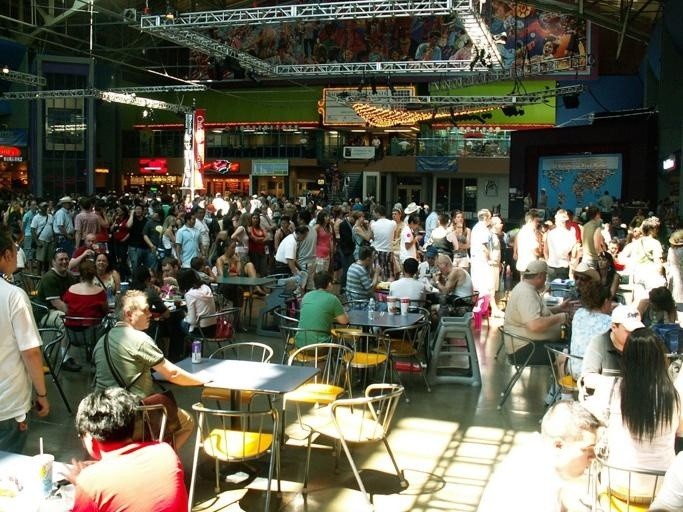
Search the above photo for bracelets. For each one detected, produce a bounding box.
[35,391,48,397]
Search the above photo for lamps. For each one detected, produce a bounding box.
[200,52,266,85]
[356,47,526,132]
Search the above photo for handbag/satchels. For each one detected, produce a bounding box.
[215,318,233,338]
[155,247,171,263]
[140,389,182,442]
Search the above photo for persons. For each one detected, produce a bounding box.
[386,258,427,342]
[1,191,508,319]
[478,259,681,512]
[426,255,474,339]
[398,139,411,151]
[57,386,189,512]
[513,189,683,326]
[372,135,380,148]
[35,249,217,453]
[190,2,584,80]
[345,245,384,338]
[1,229,50,455]
[290,271,350,385]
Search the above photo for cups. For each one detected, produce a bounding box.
[396,299,400,314]
[386,296,396,315]
[172,296,180,309]
[119,283,128,294]
[31,452,54,493]
[400,297,410,316]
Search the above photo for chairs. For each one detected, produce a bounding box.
[537,260,678,508]
[2,251,322,512]
[290,383,409,497]
[283,250,490,431]
[495,324,563,409]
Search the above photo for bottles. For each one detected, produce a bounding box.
[367,298,375,320]
[115,291,121,306]
[560,323,567,344]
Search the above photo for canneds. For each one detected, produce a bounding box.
[192,341,201,364]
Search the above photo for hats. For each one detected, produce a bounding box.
[56,196,75,206]
[522,260,554,276]
[426,247,438,258]
[393,203,403,214]
[403,202,421,214]
[669,228,683,246]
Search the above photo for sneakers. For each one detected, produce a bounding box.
[61,358,80,372]
